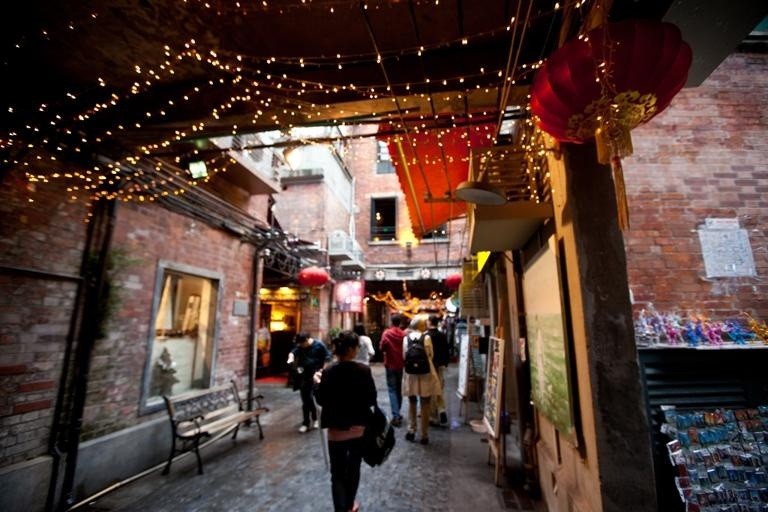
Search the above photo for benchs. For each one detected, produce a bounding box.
[163,375,270,474]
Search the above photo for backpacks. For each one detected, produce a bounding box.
[404,338,430,374]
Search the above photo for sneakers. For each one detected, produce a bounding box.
[312,419,320,429]
[391,418,402,428]
[298,425,309,432]
[421,438,428,445]
[405,432,415,441]
[428,408,448,427]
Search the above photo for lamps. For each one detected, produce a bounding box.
[456,157,528,205]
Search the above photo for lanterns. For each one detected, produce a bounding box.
[297,264,330,297]
[528,13,694,234]
[445,274,462,291]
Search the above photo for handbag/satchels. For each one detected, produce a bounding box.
[286,356,308,392]
[361,407,395,468]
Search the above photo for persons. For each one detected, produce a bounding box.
[287,330,331,436]
[309,330,378,512]
[421,315,451,432]
[351,322,375,371]
[380,312,407,429]
[397,315,436,446]
